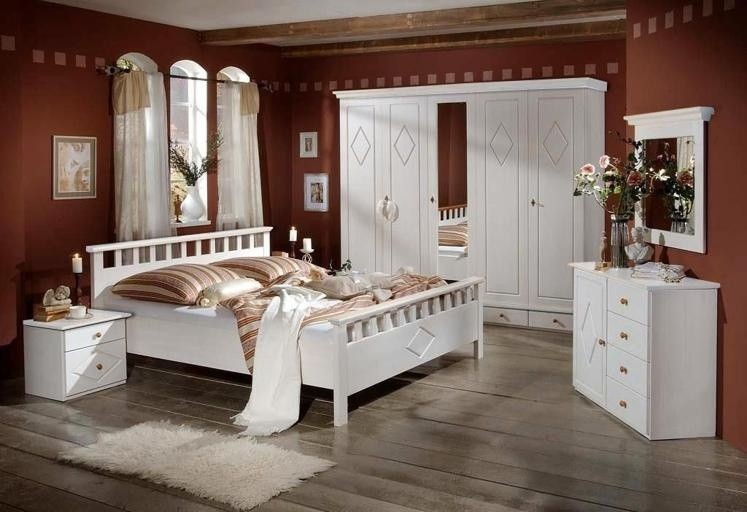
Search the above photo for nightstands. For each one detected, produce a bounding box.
[21,306,131,400]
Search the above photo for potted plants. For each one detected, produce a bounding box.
[165,127,225,223]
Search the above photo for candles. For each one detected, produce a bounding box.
[302,237,312,251]
[289,226,297,241]
[71,252,82,274]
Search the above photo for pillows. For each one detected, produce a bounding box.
[303,278,369,300]
[456,219,467,228]
[209,255,330,288]
[437,224,467,247]
[111,260,247,305]
[199,276,262,310]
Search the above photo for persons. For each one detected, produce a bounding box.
[49,285,72,305]
[314,183,321,202]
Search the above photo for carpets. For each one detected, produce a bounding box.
[59,420,337,512]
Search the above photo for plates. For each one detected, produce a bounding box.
[65,313,94,321]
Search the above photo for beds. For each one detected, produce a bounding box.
[437,203,468,277]
[84,224,485,428]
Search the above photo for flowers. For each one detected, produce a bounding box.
[649,137,697,218]
[307,257,352,283]
[562,128,675,265]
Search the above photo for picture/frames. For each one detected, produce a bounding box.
[303,172,329,213]
[51,134,98,201]
[299,131,318,159]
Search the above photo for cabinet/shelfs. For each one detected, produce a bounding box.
[332,87,437,276]
[568,262,721,440]
[477,77,608,333]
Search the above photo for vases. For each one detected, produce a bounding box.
[610,214,629,267]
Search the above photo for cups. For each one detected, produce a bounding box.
[69,306,87,318]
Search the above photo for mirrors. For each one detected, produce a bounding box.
[620,104,716,255]
[438,102,468,285]
